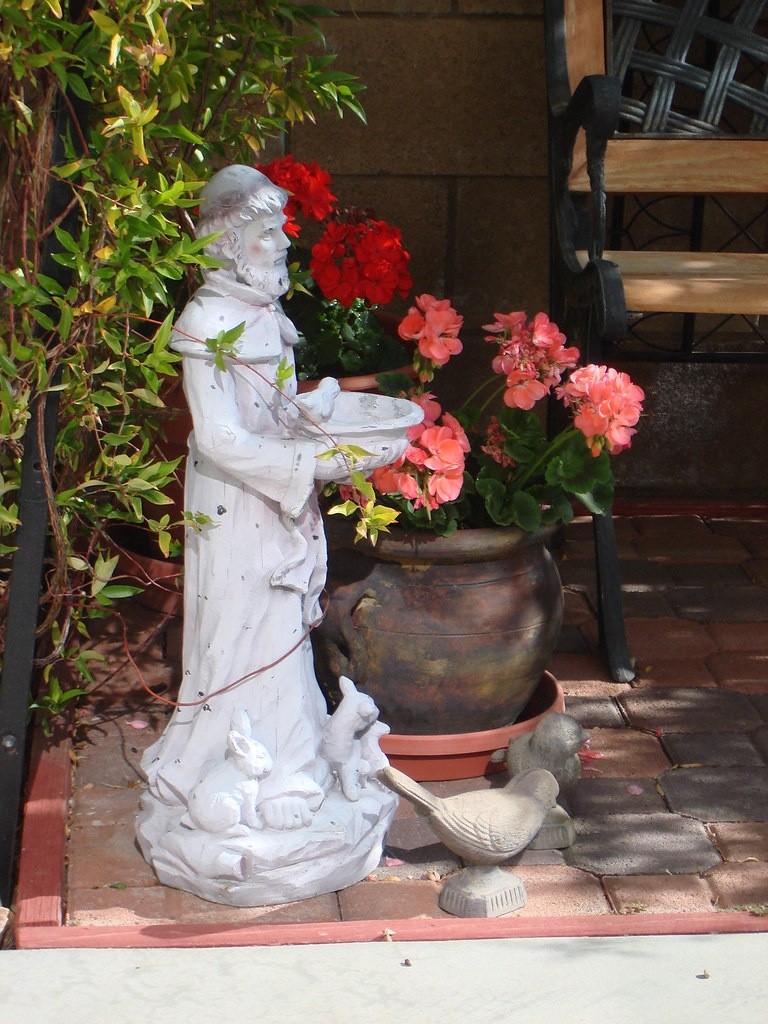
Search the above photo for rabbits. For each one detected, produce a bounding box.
[180,707,274,837]
[313,676,390,802]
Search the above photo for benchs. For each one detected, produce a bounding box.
[537,0,768,682]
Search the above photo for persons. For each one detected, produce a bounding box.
[133,165,425,907]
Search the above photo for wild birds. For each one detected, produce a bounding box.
[492,713,591,792]
[374,764,571,869]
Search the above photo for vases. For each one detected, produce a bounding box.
[93,314,417,547]
[323,521,570,726]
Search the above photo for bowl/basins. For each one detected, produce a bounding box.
[289,391,425,469]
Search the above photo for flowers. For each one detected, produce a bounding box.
[239,157,415,395]
[322,297,642,537]
